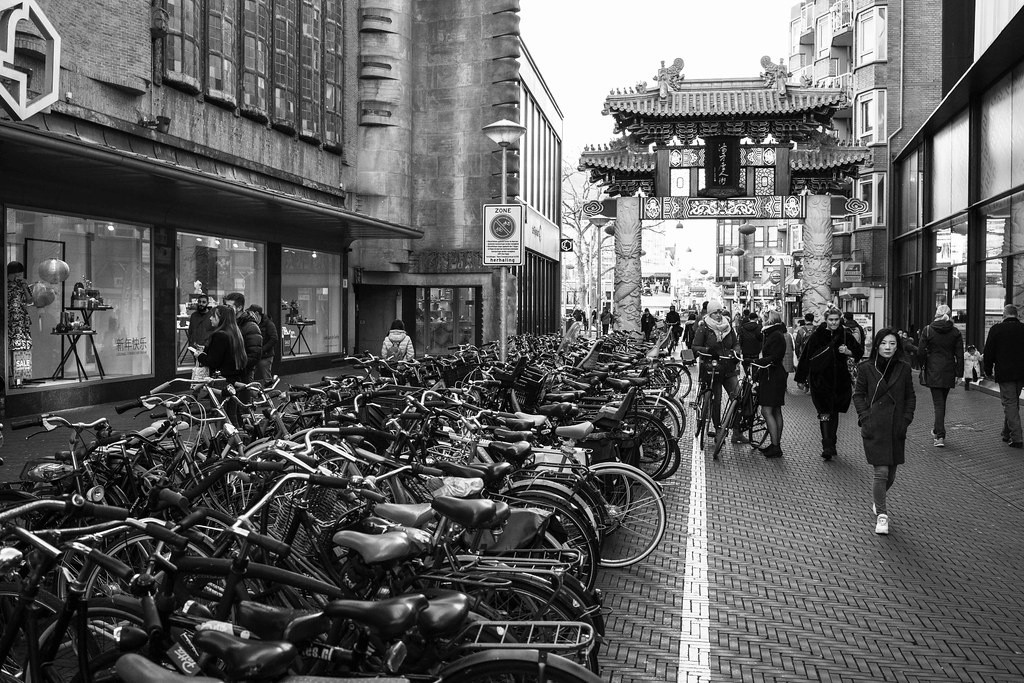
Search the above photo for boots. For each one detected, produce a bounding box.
[819,420,832,459]
[831,419,837,456]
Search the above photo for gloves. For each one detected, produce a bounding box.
[731,353,741,364]
[708,349,719,362]
[742,359,756,369]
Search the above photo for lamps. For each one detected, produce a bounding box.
[139,116,172,134]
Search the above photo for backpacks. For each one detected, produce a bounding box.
[385,338,405,362]
[799,326,816,353]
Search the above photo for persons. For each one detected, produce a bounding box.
[592,310,597,324]
[918,304,983,447]
[193,305,248,429]
[985,304,1024,447]
[7,261,34,389]
[245,304,278,407]
[600,307,613,335]
[641,308,656,340]
[665,306,680,345]
[381,320,415,385]
[795,307,919,533]
[683,300,794,456]
[223,293,263,404]
[188,296,212,364]
[582,311,588,331]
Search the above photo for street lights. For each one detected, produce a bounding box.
[590,212,612,340]
[479,117,526,363]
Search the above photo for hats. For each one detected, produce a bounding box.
[706,300,722,315]
[245,304,262,313]
[7,260,23,274]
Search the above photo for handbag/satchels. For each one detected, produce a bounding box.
[919,366,928,386]
[190,356,209,390]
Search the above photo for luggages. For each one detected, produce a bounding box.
[680,340,696,366]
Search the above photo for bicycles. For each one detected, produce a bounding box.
[0,322,693,683]
[795,352,857,398]
[707,351,770,460]
[689,350,738,452]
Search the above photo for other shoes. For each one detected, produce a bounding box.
[931,429,937,440]
[1003,437,1010,442]
[1008,441,1022,448]
[758,444,783,458]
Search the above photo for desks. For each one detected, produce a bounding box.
[51,305,112,381]
[287,322,315,354]
[176,326,197,364]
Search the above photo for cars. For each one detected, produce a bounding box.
[952,294,968,322]
[985,284,1006,316]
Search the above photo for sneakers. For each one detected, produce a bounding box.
[875,514,889,532]
[934,438,944,447]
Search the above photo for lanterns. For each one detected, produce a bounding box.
[37,257,69,293]
[605,225,615,236]
[566,263,574,269]
[732,248,745,256]
[701,270,719,287]
[739,224,756,236]
[726,267,738,282]
[26,281,55,333]
[640,250,646,256]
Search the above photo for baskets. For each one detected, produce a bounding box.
[274,483,366,563]
[456,363,484,387]
[372,395,407,418]
